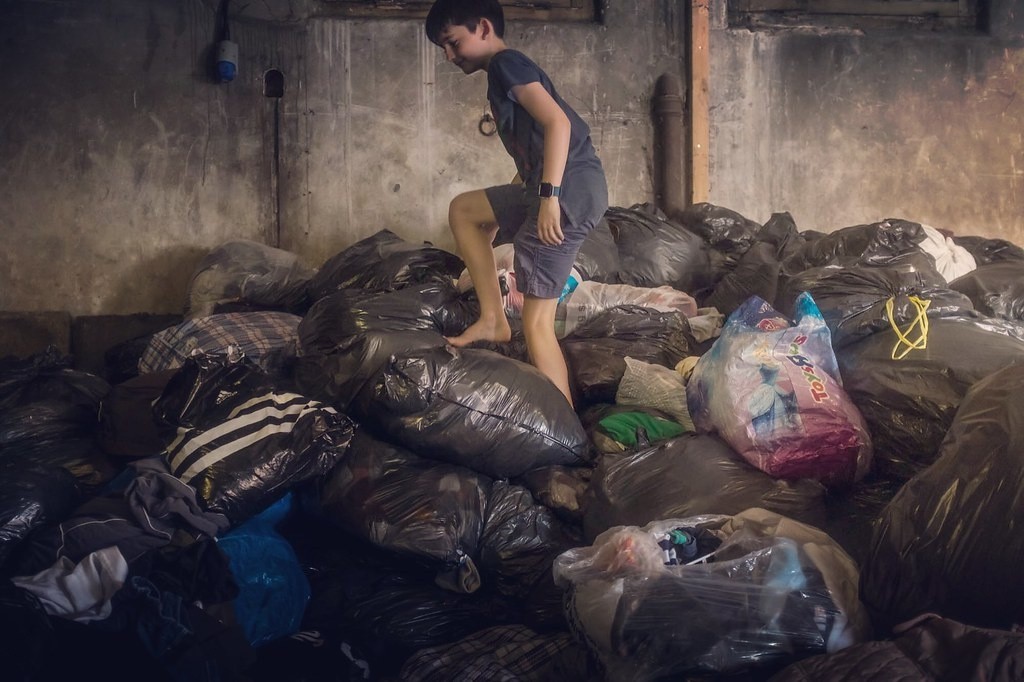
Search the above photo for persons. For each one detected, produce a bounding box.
[425,0,608,409]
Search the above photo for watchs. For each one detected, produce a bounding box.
[538,183,563,197]
